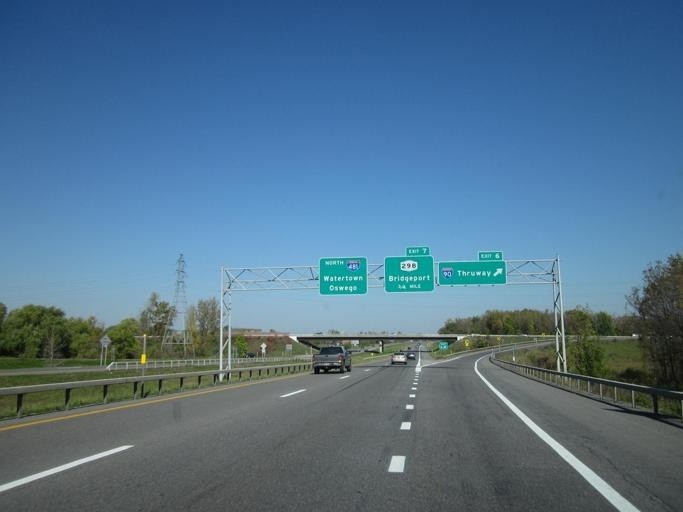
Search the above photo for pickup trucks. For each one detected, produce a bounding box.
[312,345,352,374]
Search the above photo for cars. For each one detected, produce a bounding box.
[390,351,408,365]
[407,352,416,360]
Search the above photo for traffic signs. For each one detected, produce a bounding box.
[383,255,436,294]
[319,256,368,297]
[438,260,508,286]
